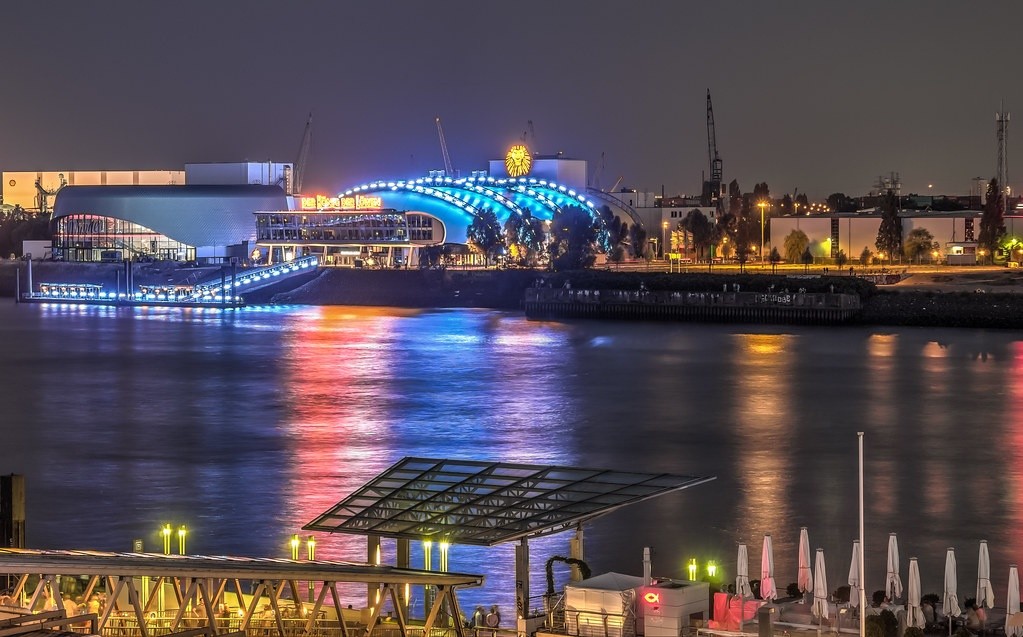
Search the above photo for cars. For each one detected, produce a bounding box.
[180,259,199,268]
[669,258,678,263]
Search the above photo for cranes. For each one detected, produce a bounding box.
[436,117,452,176]
[610,176,623,192]
[706,88,723,182]
[593,152,604,187]
[293,112,312,194]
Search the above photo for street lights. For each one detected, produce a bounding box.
[663,222,668,261]
[424,540,432,622]
[707,560,715,577]
[308,536,314,603]
[439,542,447,572]
[162,524,171,555]
[291,534,298,599]
[688,558,696,581]
[758,202,767,268]
[178,524,186,555]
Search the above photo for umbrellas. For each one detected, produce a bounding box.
[760,534,777,605]
[848,539,867,620]
[906,557,926,630]
[886,532,903,605]
[798,527,813,604]
[811,547,829,630]
[735,542,754,599]
[976,539,995,637]
[942,547,961,637]
[1005,563,1020,637]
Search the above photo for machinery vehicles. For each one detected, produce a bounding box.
[136,251,154,263]
[43,251,64,262]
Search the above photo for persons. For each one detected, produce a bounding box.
[62,595,77,616]
[192,598,206,626]
[88,596,99,613]
[965,604,987,637]
[920,601,934,623]
[222,604,230,633]
[720,584,734,593]
[880,596,889,608]
[475,605,501,628]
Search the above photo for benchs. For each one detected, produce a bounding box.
[779,602,813,625]
